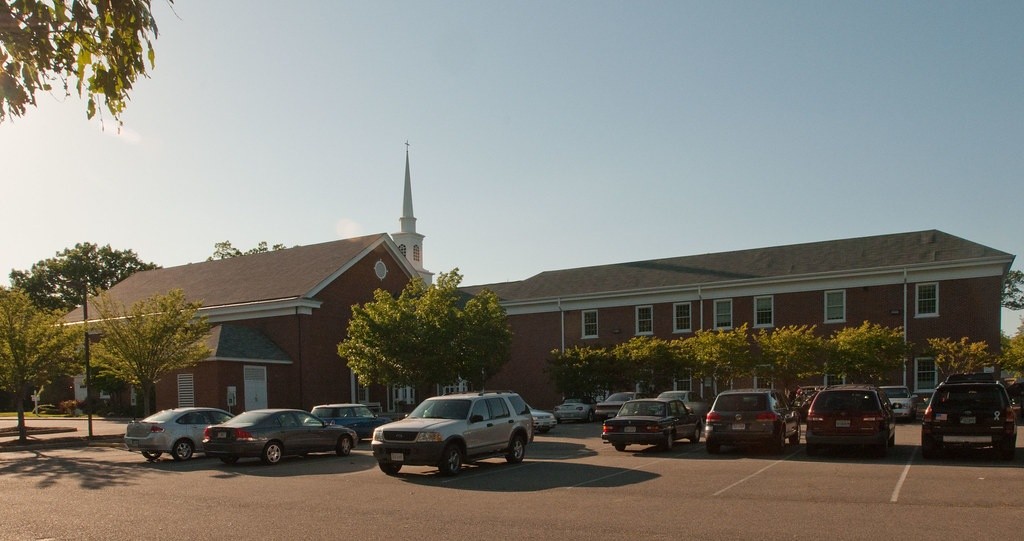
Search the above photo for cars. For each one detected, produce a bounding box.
[124,406,236,463]
[654,390,711,421]
[913,389,937,422]
[788,385,825,422]
[601,398,702,454]
[306,402,393,441]
[555,395,598,420]
[526,401,557,434]
[595,391,651,423]
[201,408,360,466]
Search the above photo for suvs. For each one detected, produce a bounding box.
[921,373,1019,461]
[805,384,903,456]
[704,388,800,455]
[878,385,919,424]
[371,389,535,477]
